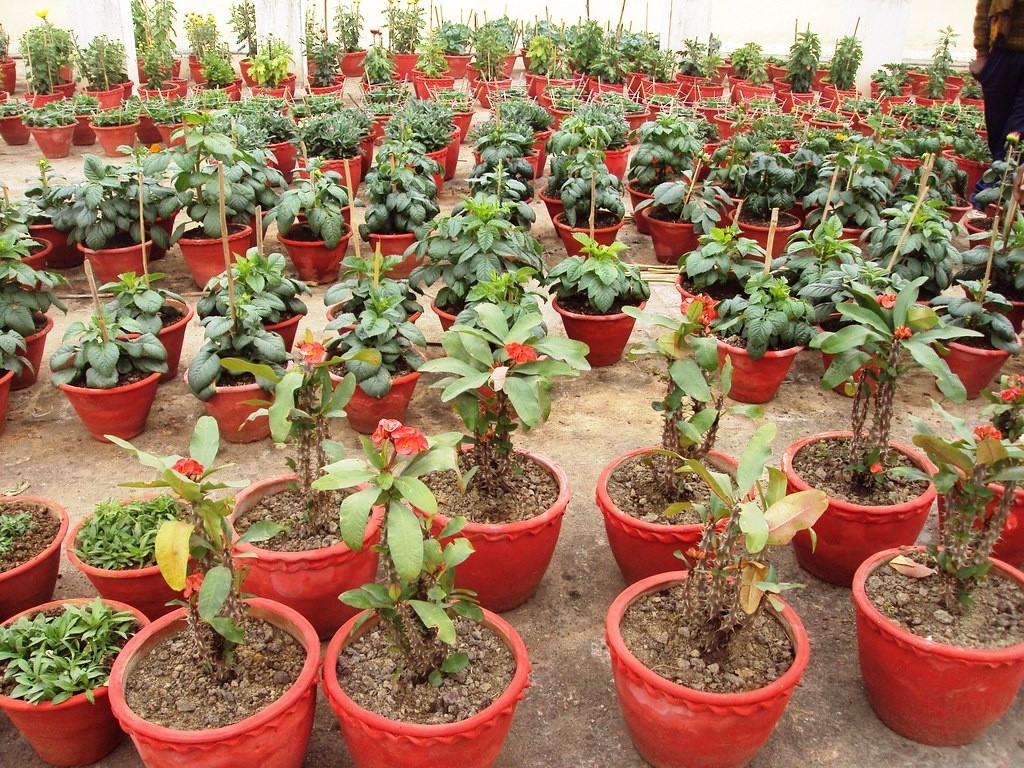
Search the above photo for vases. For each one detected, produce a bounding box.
[780,432,942,587]
[234,78,243,101]
[106,595,323,768]
[121,80,134,101]
[81,83,125,107]
[338,49,368,77]
[322,599,533,768]
[937,436,1024,569]
[188,54,198,79]
[23,89,64,108]
[301,81,344,96]
[391,73,401,80]
[393,54,420,81]
[362,80,389,91]
[225,471,388,639]
[137,83,180,102]
[37,79,76,97]
[931,333,1023,399]
[189,63,206,84]
[852,544,1024,748]
[410,440,571,611]
[192,82,237,100]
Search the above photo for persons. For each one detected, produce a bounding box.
[969,0,1024,163]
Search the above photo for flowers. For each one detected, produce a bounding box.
[134,33,179,90]
[369,32,399,77]
[99,122,1024,689]
[186,17,239,81]
[65,27,121,91]
[331,0,365,52]
[380,0,405,52]
[391,0,428,54]
[95,35,130,84]
[298,0,324,57]
[29,6,79,84]
[17,21,70,93]
[358,28,397,84]
[314,28,340,87]
[199,43,236,88]
[182,11,222,54]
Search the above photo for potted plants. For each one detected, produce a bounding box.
[799,255,922,396]
[0,597,152,768]
[540,232,652,367]
[0,231,73,389]
[70,493,208,621]
[0,0,1024,274]
[186,300,299,445]
[194,246,313,369]
[604,421,831,768]
[321,285,431,437]
[676,222,772,333]
[952,228,1024,337]
[320,254,425,337]
[595,301,757,589]
[88,268,193,382]
[0,495,69,624]
[705,272,821,404]
[48,301,171,442]
[402,195,548,352]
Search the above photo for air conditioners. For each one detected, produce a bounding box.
[0,329,35,434]
